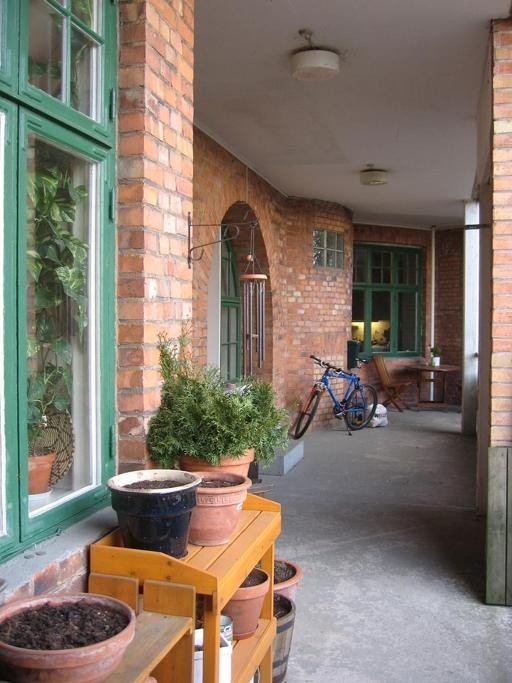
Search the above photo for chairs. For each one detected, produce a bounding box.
[371,355,411,412]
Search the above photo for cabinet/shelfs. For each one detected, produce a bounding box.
[88,488,281,682]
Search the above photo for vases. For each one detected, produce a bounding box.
[0,589,135,683]
[192,559,302,683]
[108,469,252,558]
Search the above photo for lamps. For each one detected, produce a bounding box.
[289,30,342,82]
[360,164,390,186]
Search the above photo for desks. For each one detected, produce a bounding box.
[406,365,460,408]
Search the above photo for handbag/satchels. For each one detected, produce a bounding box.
[365,403,388,427]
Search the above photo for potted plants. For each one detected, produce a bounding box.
[429,345,442,367]
[27,0,92,495]
[147,313,306,477]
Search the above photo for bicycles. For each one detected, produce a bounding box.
[294,354,378,438]
